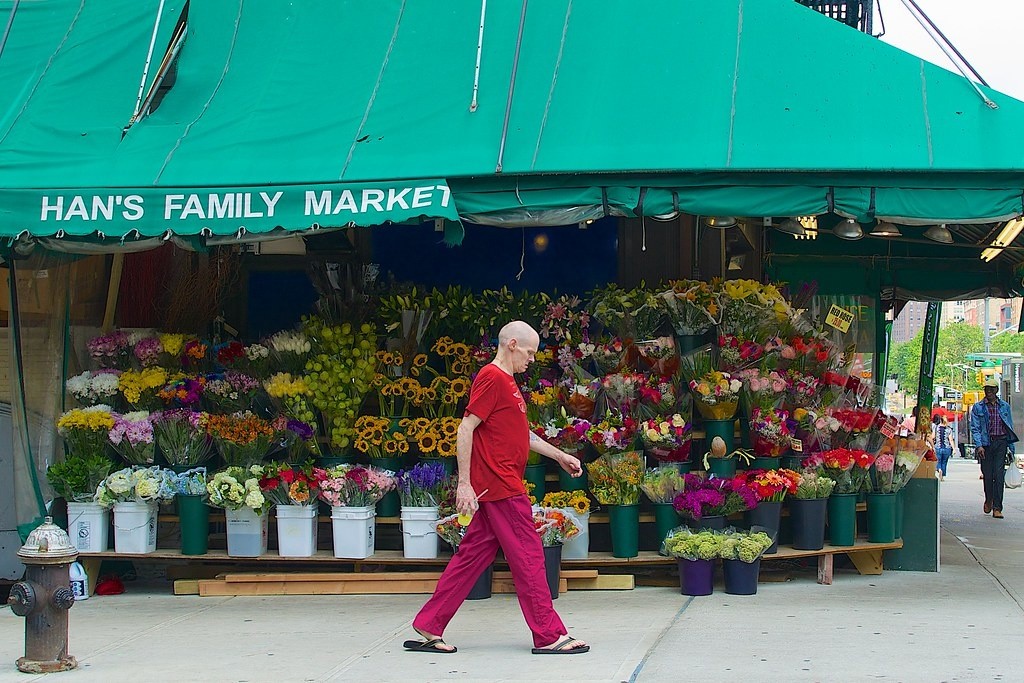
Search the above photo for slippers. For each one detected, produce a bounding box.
[403,639,457,653]
[532,637,590,654]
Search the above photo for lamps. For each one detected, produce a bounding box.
[703,215,738,229]
[922,224,954,244]
[981,216,1024,262]
[832,216,866,240]
[869,221,902,236]
[773,215,808,236]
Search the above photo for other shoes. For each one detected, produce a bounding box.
[984,497,993,513]
[939,469,945,482]
[993,508,1004,518]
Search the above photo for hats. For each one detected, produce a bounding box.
[984,378,999,389]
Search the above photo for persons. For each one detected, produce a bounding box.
[403,318,592,654]
[959,417,970,457]
[931,413,956,483]
[970,378,1020,518]
[899,406,918,434]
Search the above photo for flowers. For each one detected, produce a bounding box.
[55,276,930,563]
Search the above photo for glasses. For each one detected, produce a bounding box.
[984,387,997,393]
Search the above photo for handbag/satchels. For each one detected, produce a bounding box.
[1004,462,1021,489]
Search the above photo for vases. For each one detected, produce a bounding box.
[824,493,860,546]
[742,501,783,555]
[453,545,493,600]
[523,464,547,504]
[417,455,454,481]
[175,493,208,555]
[316,455,350,517]
[720,556,762,595]
[365,456,404,517]
[606,502,640,557]
[556,463,589,496]
[697,513,729,531]
[166,464,200,476]
[651,503,685,556]
[543,541,564,599]
[865,493,897,543]
[674,556,716,596]
[786,497,828,550]
[702,417,738,456]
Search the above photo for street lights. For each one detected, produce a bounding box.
[945,364,976,392]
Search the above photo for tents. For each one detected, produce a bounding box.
[930,407,964,424]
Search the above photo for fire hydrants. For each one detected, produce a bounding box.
[7,516,83,674]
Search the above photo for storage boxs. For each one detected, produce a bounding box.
[910,461,938,479]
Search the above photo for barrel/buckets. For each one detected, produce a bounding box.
[111,501,160,554]
[65,501,109,553]
[531,505,590,560]
[276,501,319,557]
[329,504,378,560]
[400,505,441,559]
[225,504,268,557]
